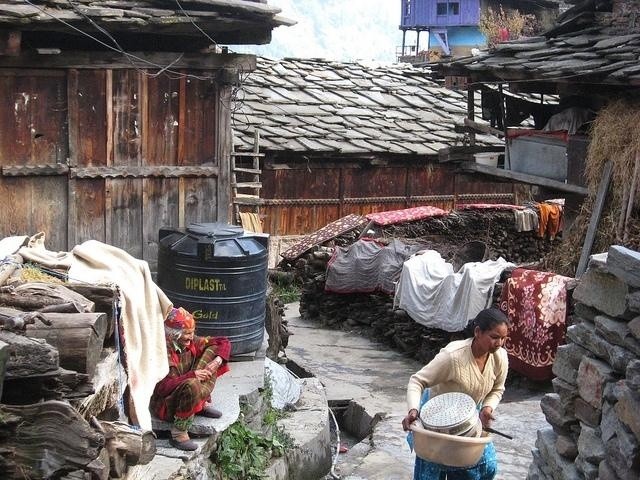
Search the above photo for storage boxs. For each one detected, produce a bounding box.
[505,128,591,186]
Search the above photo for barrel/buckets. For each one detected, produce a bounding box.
[474,152,499,169]
[158,221,270,356]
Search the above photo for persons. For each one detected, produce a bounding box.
[403,307,509,480]
[148,305,232,451]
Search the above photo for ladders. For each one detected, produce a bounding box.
[230,128,265,228]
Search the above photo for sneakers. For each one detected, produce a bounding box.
[168,433,198,451]
[196,406,222,418]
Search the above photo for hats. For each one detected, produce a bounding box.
[165,306,196,330]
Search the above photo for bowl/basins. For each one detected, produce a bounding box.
[407,421,495,466]
